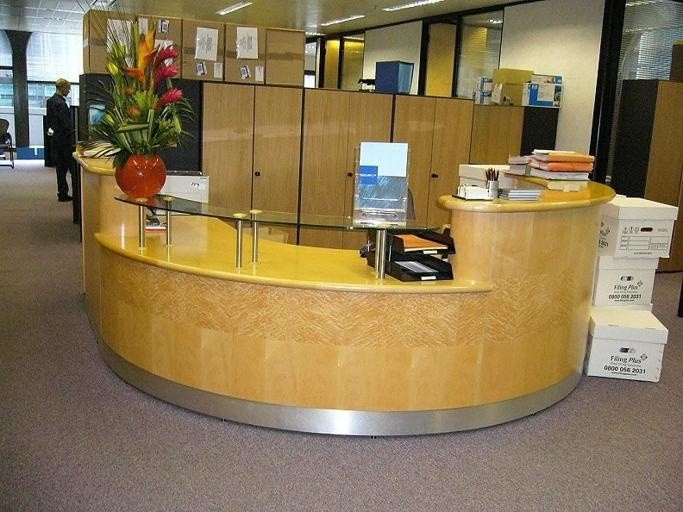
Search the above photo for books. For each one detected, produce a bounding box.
[498,147,596,202]
[144,217,166,233]
[391,232,448,252]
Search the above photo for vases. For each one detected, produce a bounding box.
[113,155,167,201]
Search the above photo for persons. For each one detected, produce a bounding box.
[41,77,79,203]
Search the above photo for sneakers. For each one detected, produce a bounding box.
[57,193,73,202]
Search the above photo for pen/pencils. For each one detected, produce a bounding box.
[486,168,499,181]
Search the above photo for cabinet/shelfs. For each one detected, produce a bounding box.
[469,103,524,166]
[298,89,394,252]
[200,81,303,249]
[392,91,474,237]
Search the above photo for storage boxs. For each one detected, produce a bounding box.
[470,68,565,110]
[581,195,678,385]
[81,8,306,87]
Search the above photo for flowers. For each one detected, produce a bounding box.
[76,1,198,165]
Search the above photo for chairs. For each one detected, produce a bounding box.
[0,117,14,168]
